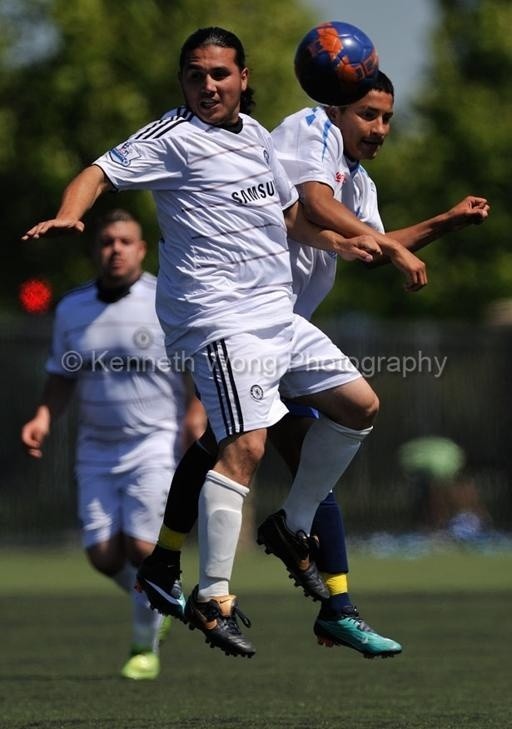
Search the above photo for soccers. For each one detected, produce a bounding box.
[294,22,378,108]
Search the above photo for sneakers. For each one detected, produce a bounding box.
[134,545,187,620]
[313,608,403,659]
[255,507,331,602]
[120,616,174,681]
[183,583,257,659]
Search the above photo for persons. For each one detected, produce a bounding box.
[15,25,387,658]
[131,61,493,660]
[15,207,207,684]
